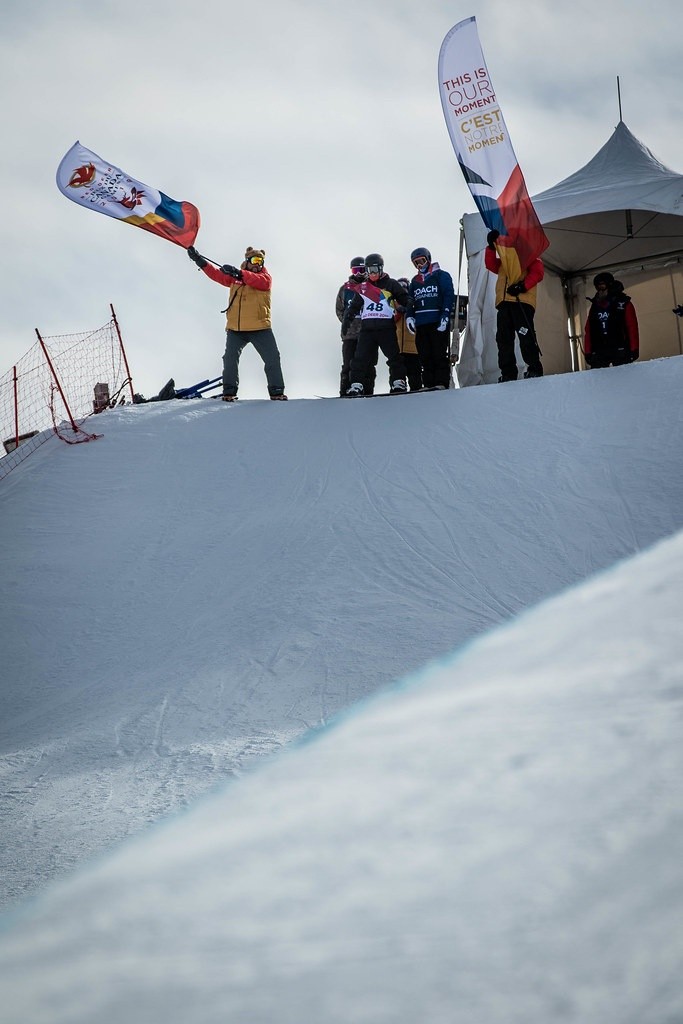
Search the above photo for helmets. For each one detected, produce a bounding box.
[410,247,432,274]
[364,253,384,267]
[349,256,366,269]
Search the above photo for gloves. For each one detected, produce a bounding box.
[486,229,500,250]
[506,280,527,297]
[436,317,449,331]
[584,352,595,366]
[672,304,683,317]
[629,350,640,361]
[342,318,351,337]
[405,317,416,335]
[219,264,243,281]
[187,245,207,268]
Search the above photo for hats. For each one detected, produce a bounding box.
[397,277,410,290]
[593,272,614,287]
[240,247,266,270]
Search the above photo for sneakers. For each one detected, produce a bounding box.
[391,379,406,392]
[345,383,364,396]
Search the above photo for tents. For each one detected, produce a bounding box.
[449,121,683,389]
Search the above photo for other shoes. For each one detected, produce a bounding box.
[222,395,239,402]
[270,394,288,401]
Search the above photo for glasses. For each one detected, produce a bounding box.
[367,264,384,274]
[244,255,264,266]
[397,281,408,288]
[351,266,367,275]
[595,277,612,291]
[412,255,431,269]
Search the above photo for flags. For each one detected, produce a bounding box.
[437,17,550,285]
[55,142,200,248]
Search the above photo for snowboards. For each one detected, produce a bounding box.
[313,385,445,399]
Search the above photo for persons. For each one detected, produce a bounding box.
[335,257,367,396]
[485,230,544,383]
[187,246,288,401]
[404,248,454,390]
[583,272,640,370]
[342,253,408,394]
[393,278,423,390]
